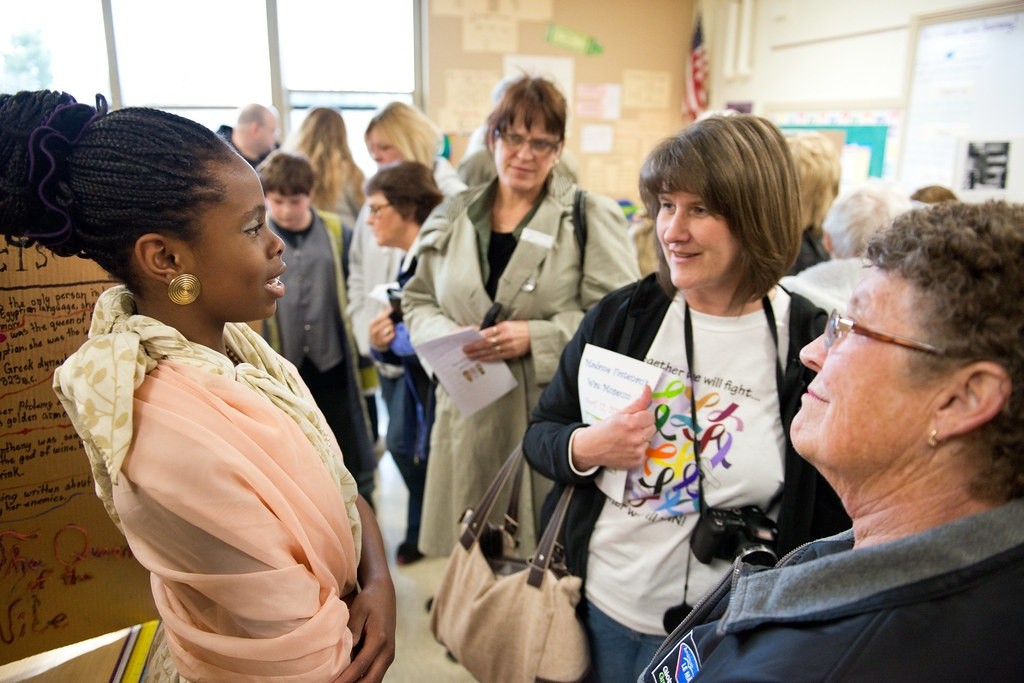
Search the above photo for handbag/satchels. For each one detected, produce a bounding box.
[427,440,592,683]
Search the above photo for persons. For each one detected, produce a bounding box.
[261,108,367,220]
[399,79,640,559]
[786,130,956,320]
[218,103,280,168]
[348,102,468,566]
[522,115,852,683]
[637,196,1024,683]
[246,152,377,513]
[0,91,396,683]
[458,74,578,187]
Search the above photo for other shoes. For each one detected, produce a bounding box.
[397,540,426,564]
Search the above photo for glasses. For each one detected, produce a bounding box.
[824,310,941,358]
[495,129,560,156]
[369,203,393,218]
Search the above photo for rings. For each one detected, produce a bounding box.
[496,345,500,351]
[492,338,497,344]
[384,329,389,334]
[493,327,496,335]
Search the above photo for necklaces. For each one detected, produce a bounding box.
[225,346,242,365]
